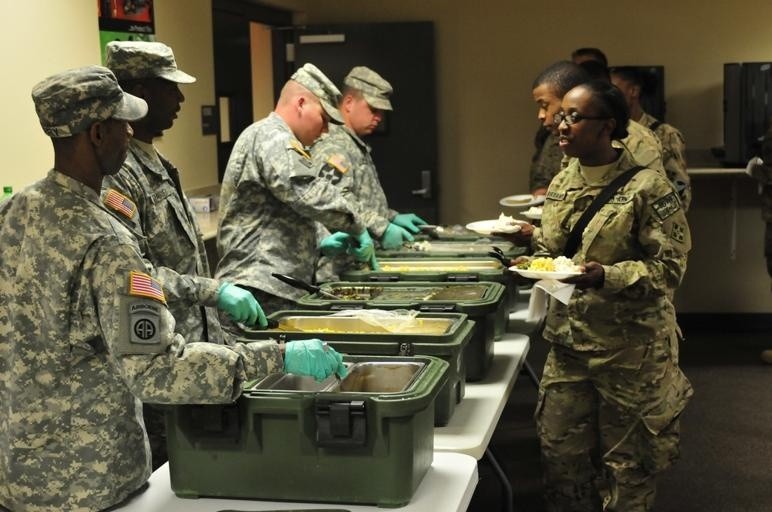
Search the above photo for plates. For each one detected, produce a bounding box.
[497,190,547,208]
[463,216,529,238]
[521,209,543,220]
[508,264,587,284]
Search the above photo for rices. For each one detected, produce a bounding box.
[552,255,576,273]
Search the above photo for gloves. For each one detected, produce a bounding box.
[352,230,376,270]
[320,232,352,256]
[380,223,414,250]
[392,213,428,233]
[284,338,347,382]
[217,281,268,329]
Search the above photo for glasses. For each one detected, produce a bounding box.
[554,113,610,125]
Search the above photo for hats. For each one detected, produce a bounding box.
[344,66,394,111]
[32,67,149,138]
[290,63,345,125]
[106,41,196,84]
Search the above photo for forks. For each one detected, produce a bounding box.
[486,244,508,268]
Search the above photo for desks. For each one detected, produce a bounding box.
[431,331,541,512]
[103,449,481,512]
[503,289,548,345]
[194,210,218,244]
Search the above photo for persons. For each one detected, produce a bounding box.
[304,66,434,287]
[0,66,348,512]
[510,81,693,512]
[213,61,377,342]
[744,127,772,365]
[489,47,693,260]
[98,39,268,475]
